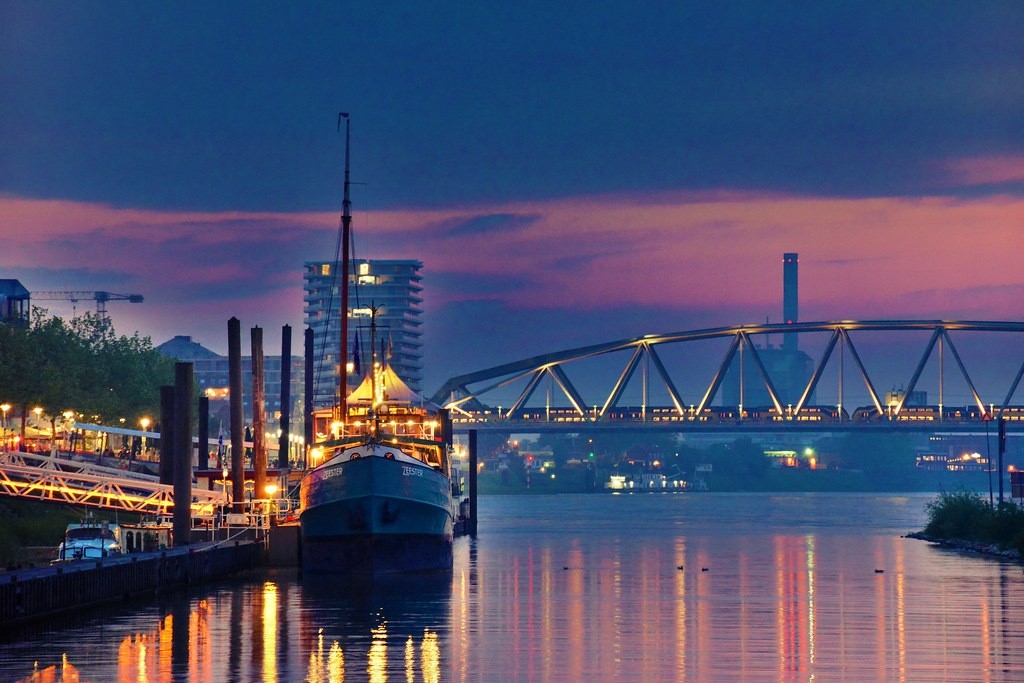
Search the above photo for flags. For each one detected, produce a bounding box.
[218,419,224,454]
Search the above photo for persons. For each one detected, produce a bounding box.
[94,447,115,458]
[118,449,130,459]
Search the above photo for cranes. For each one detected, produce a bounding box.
[28,289,144,330]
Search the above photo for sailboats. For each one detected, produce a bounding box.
[296,111,455,554]
